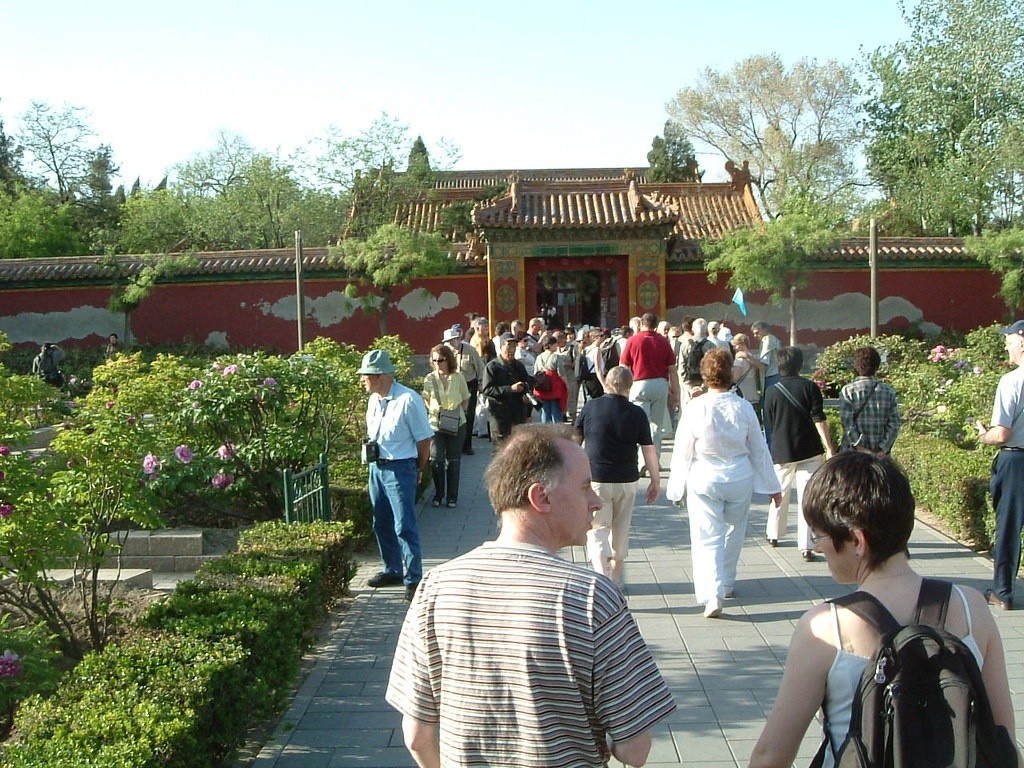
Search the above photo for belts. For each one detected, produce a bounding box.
[1001,447,1024,451]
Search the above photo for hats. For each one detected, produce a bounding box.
[707,321,721,331]
[999,320,1024,335]
[500,332,516,341]
[442,329,460,342]
[356,350,396,375]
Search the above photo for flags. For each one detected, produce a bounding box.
[733,288,747,316]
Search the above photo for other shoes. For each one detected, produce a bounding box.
[765,534,778,548]
[638,464,648,477]
[448,501,456,507]
[431,499,440,507]
[704,598,722,619]
[367,572,403,588]
[983,589,1013,611]
[405,583,418,601]
[467,450,473,455]
[802,551,816,562]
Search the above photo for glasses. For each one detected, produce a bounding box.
[432,359,446,363]
[672,336,679,338]
[809,528,865,544]
[523,338,530,343]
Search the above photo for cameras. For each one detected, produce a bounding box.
[361,439,377,464]
[521,382,530,392]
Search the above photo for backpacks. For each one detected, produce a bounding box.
[601,336,620,378]
[534,352,553,392]
[681,338,709,386]
[809,578,1019,768]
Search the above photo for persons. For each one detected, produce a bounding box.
[355,349,434,601]
[481,332,532,451]
[421,345,471,509]
[975,319,1024,610]
[622,313,679,477]
[452,312,643,439]
[384,422,677,768]
[666,348,782,618]
[838,347,901,459]
[32,343,66,392]
[748,450,1015,768]
[105,333,123,360]
[442,328,483,455]
[656,314,783,450]
[571,367,660,591]
[765,346,836,562]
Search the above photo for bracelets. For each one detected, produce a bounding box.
[746,355,752,361]
[417,468,423,472]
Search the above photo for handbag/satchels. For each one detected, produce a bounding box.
[438,409,461,436]
[583,371,604,400]
